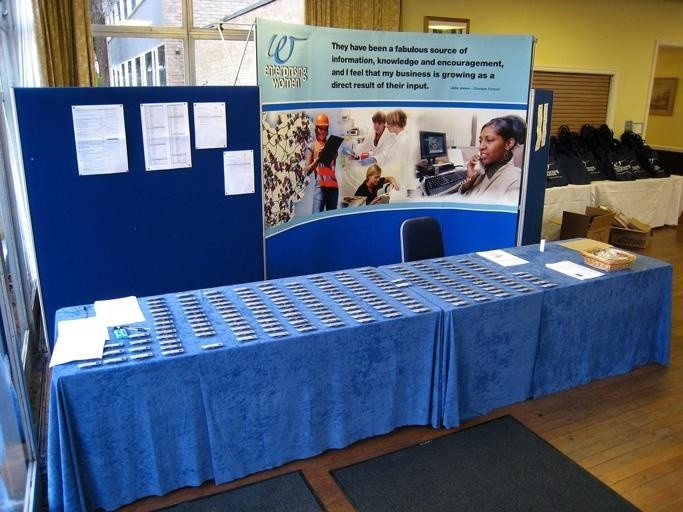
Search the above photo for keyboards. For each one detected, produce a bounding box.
[425,169,467,196]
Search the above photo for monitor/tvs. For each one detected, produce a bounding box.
[419,131,447,165]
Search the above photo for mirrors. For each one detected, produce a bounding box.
[642,42,682,153]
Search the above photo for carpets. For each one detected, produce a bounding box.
[151,468,329,512]
[329,415,641,512]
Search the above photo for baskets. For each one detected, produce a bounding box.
[581,247,636,272]
[609,223,652,249]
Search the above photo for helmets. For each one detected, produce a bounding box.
[315,114,329,126]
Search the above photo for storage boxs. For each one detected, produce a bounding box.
[551,207,616,244]
[610,218,652,249]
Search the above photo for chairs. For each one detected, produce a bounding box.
[400,217,444,263]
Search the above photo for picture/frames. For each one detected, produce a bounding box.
[649,78,679,116]
[424,16,470,33]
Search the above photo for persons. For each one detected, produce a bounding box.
[338,110,395,168]
[379,108,419,191]
[303,111,339,216]
[456,114,527,207]
[353,164,401,206]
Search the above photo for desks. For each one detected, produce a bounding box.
[541,174,683,242]
[47,266,444,512]
[377,237,673,429]
